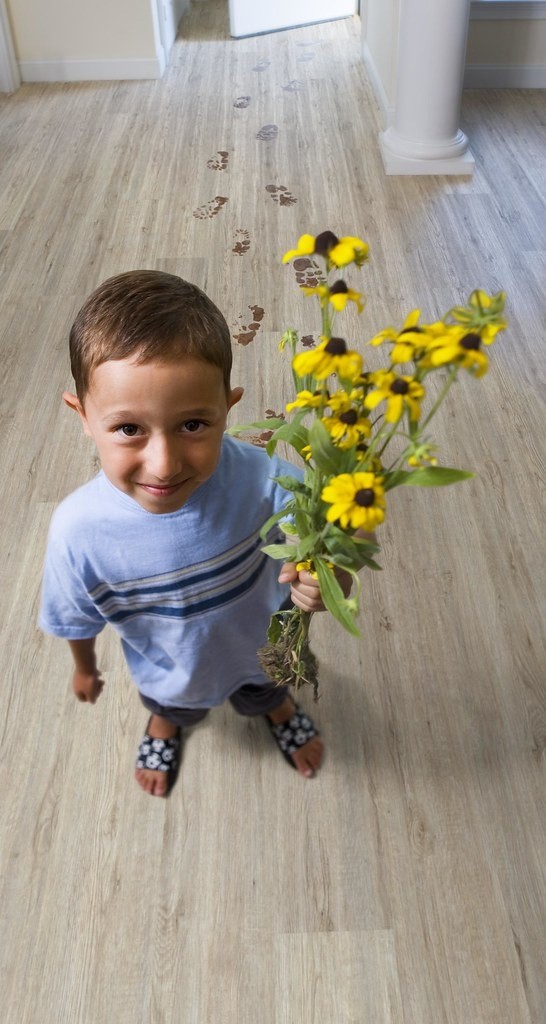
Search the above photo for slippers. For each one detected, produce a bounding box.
[136,714,182,797]
[264,696,321,770]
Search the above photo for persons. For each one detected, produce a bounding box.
[38,270,379,796]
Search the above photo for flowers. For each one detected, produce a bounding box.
[222,228,508,706]
